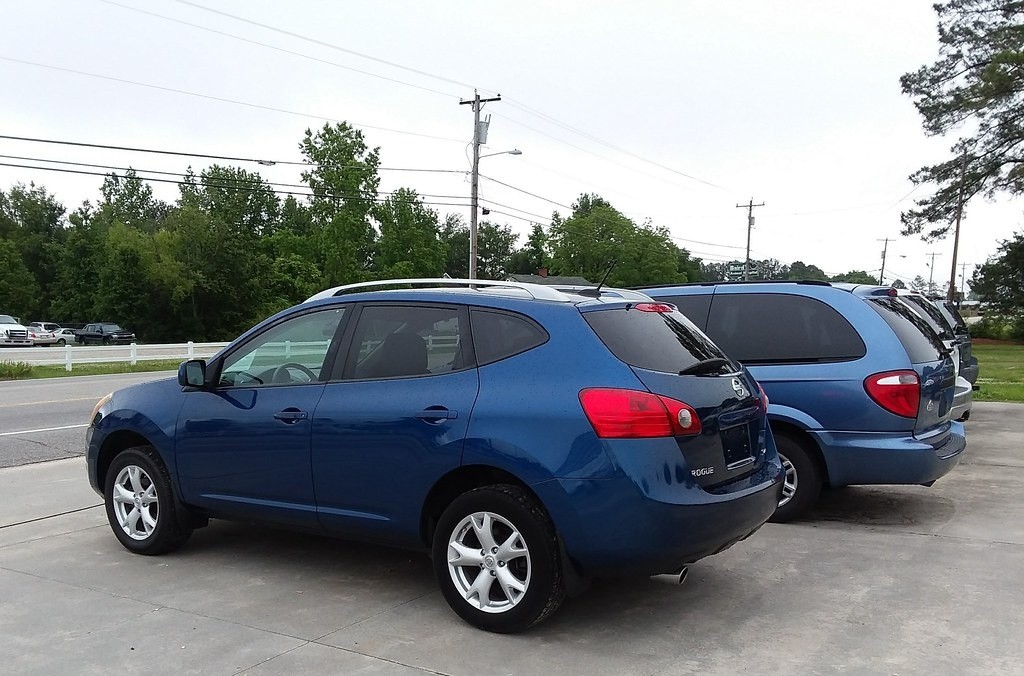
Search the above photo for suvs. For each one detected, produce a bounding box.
[626,281,981,524]
[0,315,33,346]
[86,279,785,636]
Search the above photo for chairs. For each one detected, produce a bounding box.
[381,332,430,376]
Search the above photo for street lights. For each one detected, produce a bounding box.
[469,150,523,290]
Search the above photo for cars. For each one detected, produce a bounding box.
[74,323,137,346]
[22,322,84,347]
